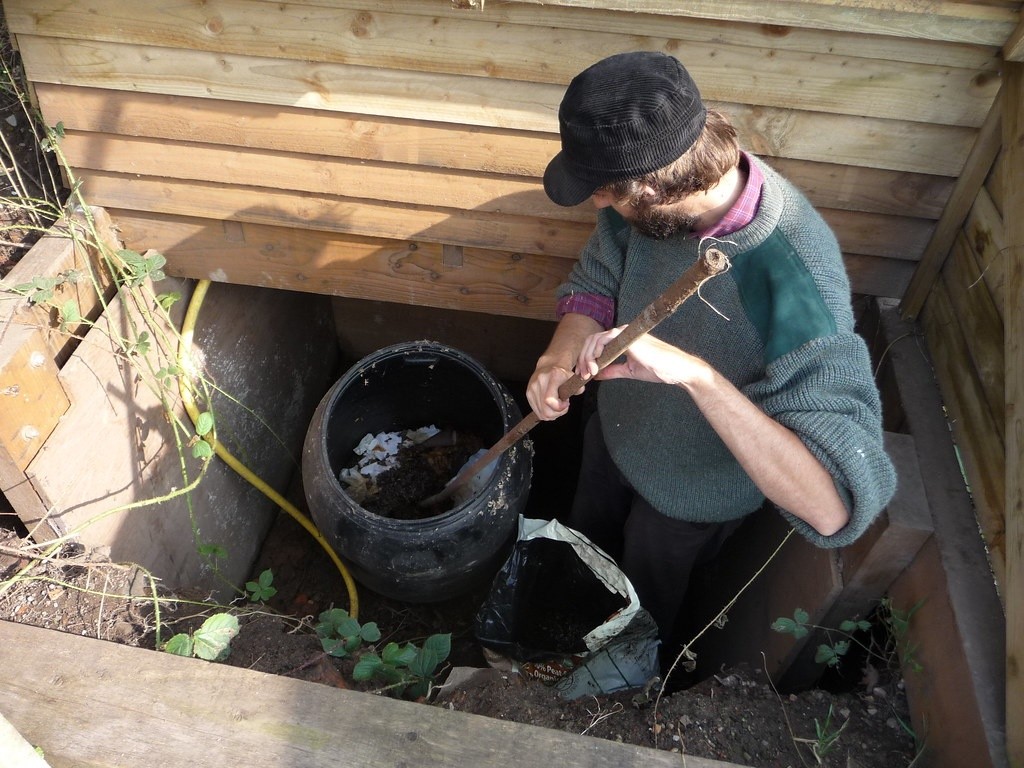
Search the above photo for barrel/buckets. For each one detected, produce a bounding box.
[301,341,533,605]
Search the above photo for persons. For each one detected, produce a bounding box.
[526,51,898,673]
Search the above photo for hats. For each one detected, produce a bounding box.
[543,52,706,207]
